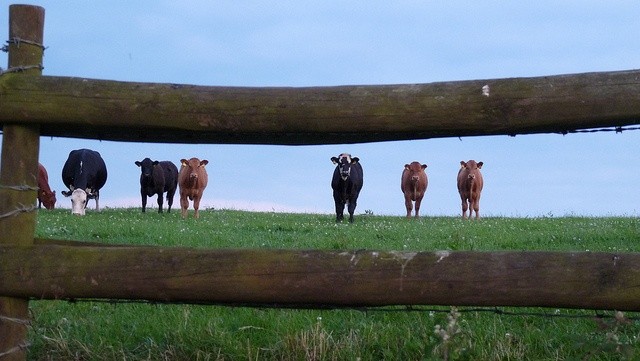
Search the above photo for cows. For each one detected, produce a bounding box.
[401,162,429,219]
[135,158,179,214]
[61,148,109,216]
[330,154,365,224]
[457,160,485,221]
[36,162,57,210]
[178,156,210,221]
